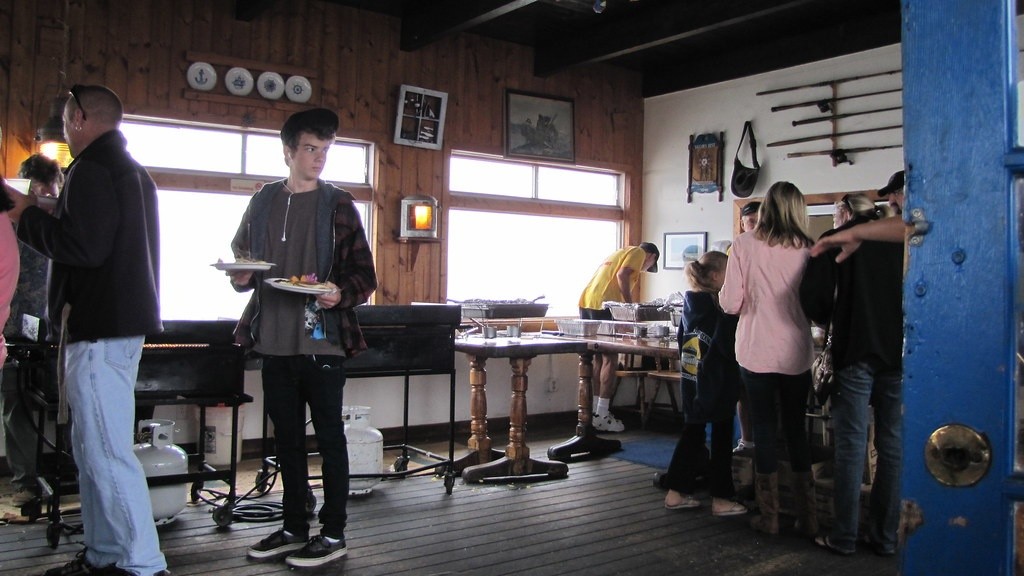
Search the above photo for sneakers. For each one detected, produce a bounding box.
[731,441,755,454]
[591,412,625,434]
[288,536,346,567]
[45,557,171,575]
[818,527,895,559]
[247,527,307,558]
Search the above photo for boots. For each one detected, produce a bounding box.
[750,472,781,536]
[794,467,820,535]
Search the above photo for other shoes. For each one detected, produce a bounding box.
[12,487,41,508]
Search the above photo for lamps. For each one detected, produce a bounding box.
[396,192,441,242]
[36,95,73,163]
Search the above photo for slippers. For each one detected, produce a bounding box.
[665,495,700,510]
[711,502,747,517]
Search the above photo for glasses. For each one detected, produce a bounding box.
[841,194,852,216]
[69,84,88,120]
[893,190,904,196]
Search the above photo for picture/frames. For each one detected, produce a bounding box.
[500,85,578,167]
[663,229,709,273]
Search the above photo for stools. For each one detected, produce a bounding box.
[608,369,650,432]
[646,369,685,416]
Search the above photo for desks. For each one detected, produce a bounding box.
[435,331,589,485]
[539,328,680,460]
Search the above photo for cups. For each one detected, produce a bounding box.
[483,326,498,339]
[507,325,521,337]
[4,178,31,196]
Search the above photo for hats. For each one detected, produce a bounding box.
[644,242,659,273]
[742,201,760,214]
[877,170,905,196]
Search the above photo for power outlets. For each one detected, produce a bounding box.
[546,376,560,394]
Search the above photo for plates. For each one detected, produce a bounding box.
[37,194,59,205]
[262,278,331,296]
[212,262,276,270]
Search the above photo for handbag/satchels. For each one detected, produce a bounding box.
[730,119,761,197]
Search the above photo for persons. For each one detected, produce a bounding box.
[579,241,661,437]
[4,83,172,576]
[650,169,909,561]
[216,104,380,568]
[1,154,67,511]
[1,147,21,381]
